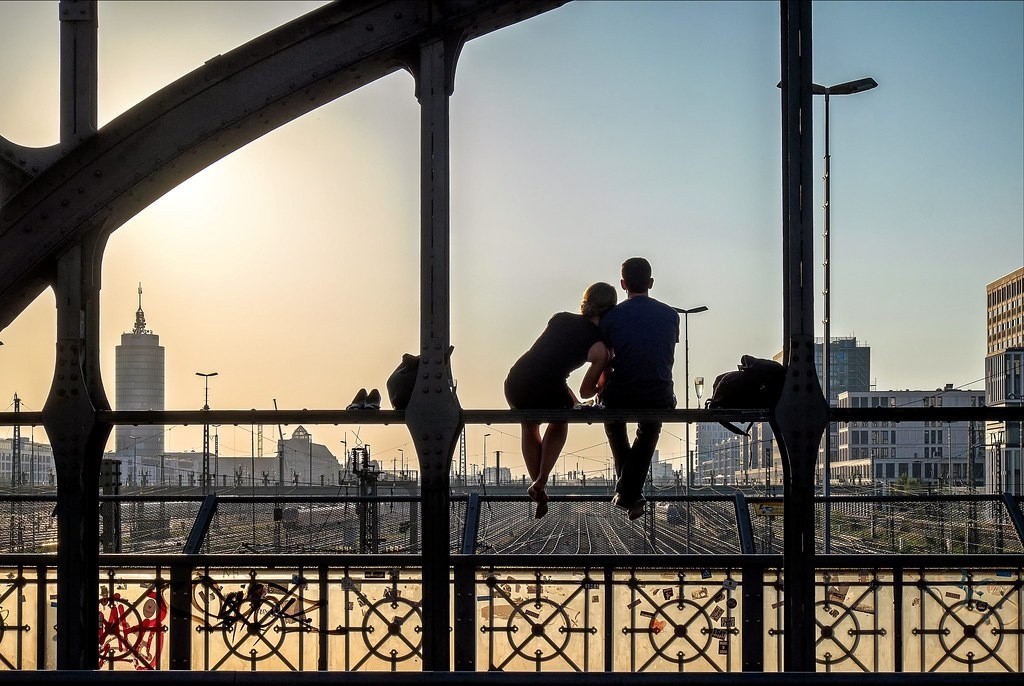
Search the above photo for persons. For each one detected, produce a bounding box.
[504,281,613,518]
[597,257,679,518]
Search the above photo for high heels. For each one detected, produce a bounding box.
[361,389,381,410]
[346,389,368,411]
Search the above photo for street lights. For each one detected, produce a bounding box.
[669,305,711,552]
[775,69,881,607]
[193,370,218,493]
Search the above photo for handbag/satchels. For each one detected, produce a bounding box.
[387,353,420,410]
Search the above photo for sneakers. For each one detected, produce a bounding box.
[628,496,647,521]
[610,492,632,512]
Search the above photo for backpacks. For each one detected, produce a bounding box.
[704,354,787,439]
